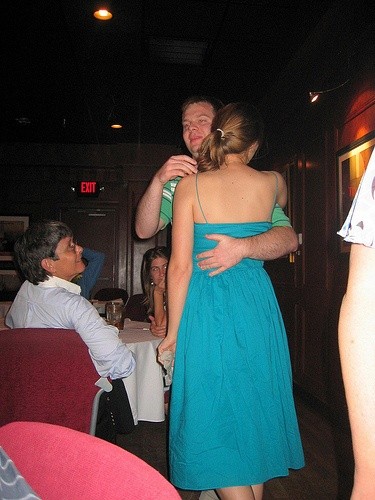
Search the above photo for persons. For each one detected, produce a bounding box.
[71,248,106,301]
[4,220,137,436]
[156,102,306,500]
[134,95,297,276]
[336,147,375,500]
[141,246,171,337]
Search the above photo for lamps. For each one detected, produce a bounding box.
[309,78,351,103]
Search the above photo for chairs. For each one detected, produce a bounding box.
[94,288,153,323]
[0,328,112,437]
[0,421,182,500]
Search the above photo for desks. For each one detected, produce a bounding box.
[118,320,171,425]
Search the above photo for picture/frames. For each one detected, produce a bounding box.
[0,212,33,265]
[335,129,375,256]
[0,267,24,330]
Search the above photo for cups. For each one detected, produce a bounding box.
[105,301,126,331]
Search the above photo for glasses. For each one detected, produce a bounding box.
[54,238,79,255]
